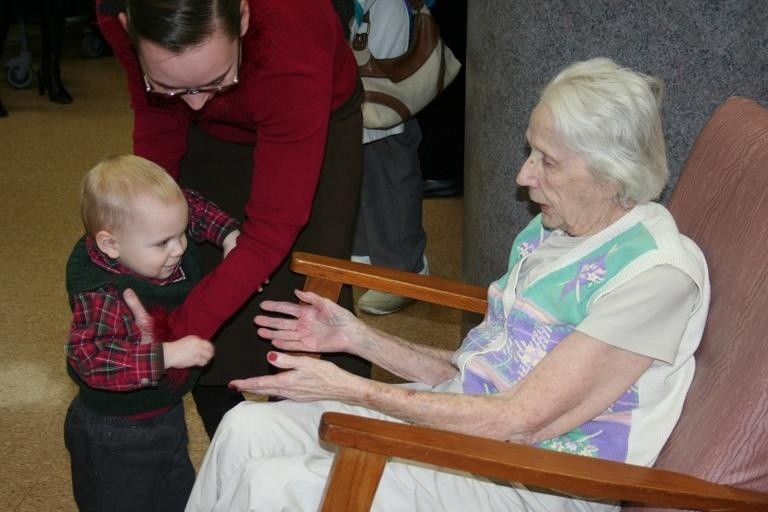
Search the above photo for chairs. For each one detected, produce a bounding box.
[278,94,767,507]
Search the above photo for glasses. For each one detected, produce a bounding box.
[143,36,242,97]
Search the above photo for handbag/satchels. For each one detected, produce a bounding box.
[348,0,463,130]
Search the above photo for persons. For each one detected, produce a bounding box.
[96,0,373,444]
[332,0,429,315]
[416,0,467,199]
[63,152,270,512]
[0,0,93,119]
[184,55,711,512]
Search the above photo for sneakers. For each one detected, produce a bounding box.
[356,254,429,316]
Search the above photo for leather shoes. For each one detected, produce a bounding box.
[422,180,462,198]
